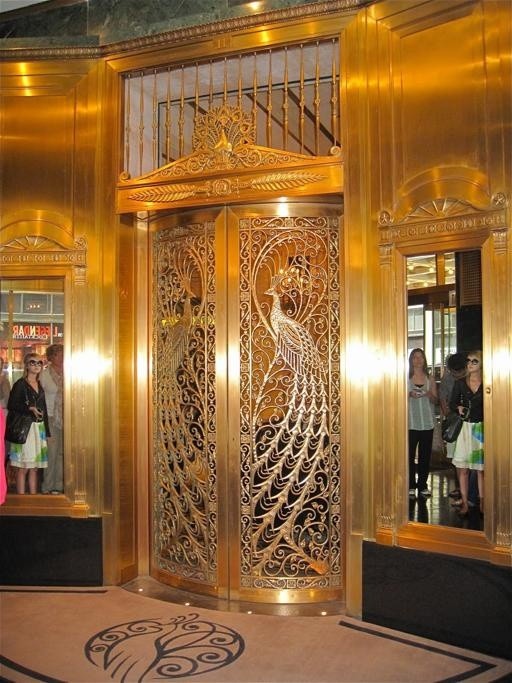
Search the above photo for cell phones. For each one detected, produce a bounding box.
[37,408,43,419]
[460,407,469,419]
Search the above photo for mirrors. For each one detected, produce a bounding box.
[397,234,497,550]
[0,268,76,508]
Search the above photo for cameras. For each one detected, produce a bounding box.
[415,392,424,397]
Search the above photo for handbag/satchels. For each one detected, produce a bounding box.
[5,412,33,444]
[442,416,462,442]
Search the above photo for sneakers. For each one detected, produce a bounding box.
[449,490,475,515]
[420,490,431,495]
[409,489,416,495]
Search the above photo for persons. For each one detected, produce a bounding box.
[0,356,10,407]
[442,352,479,498]
[5,352,50,495]
[39,343,63,495]
[451,349,484,515]
[407,348,438,497]
[1,404,8,507]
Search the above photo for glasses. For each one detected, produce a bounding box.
[466,359,478,365]
[30,359,43,367]
